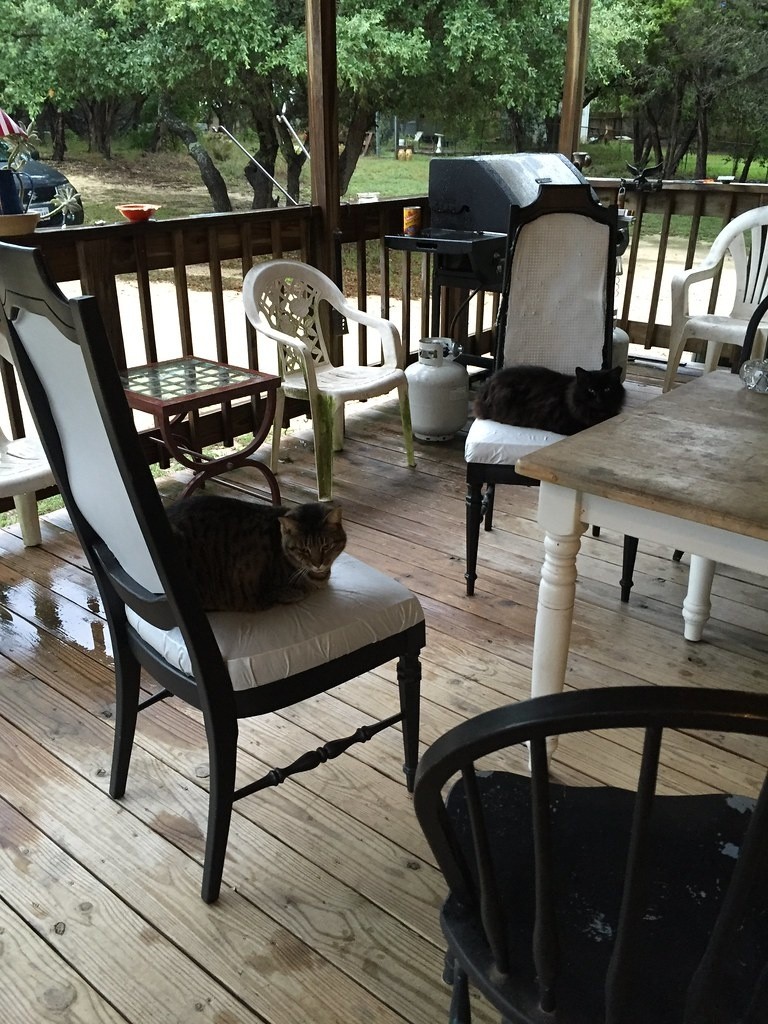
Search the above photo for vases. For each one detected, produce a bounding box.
[0,170,40,237]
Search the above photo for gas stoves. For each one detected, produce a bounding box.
[382,153,640,295]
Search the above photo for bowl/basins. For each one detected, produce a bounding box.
[114,203,162,224]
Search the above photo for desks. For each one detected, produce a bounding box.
[515,369,767,771]
[119,356,281,506]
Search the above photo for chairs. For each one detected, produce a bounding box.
[0,241,426,904]
[464,183,639,603]
[0,429,59,546]
[662,205,768,393]
[242,259,416,502]
[413,687,768,1023]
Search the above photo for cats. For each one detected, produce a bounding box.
[470,364,629,440]
[161,498,346,612]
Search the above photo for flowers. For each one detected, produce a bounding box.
[7,90,82,224]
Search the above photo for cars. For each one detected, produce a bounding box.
[0,137,84,228]
